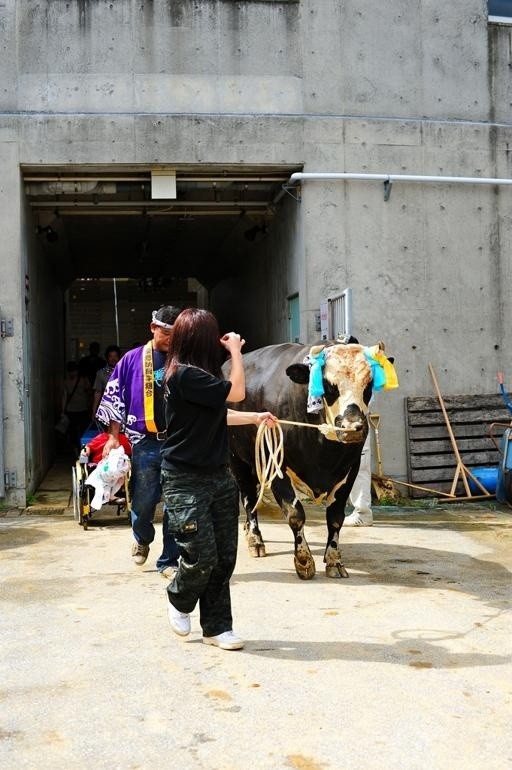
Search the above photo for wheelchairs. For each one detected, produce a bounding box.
[69,458,135,530]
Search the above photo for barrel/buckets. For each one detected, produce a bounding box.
[468,468,498,496]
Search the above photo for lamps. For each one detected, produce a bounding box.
[241,220,269,241]
[32,224,59,245]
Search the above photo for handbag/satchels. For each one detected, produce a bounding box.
[79,430,104,449]
[52,412,71,436]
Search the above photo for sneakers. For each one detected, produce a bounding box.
[164,585,192,636]
[160,565,181,582]
[130,538,152,565]
[341,507,374,528]
[201,628,247,651]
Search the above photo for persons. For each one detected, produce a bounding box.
[157,306,282,651]
[51,338,146,458]
[79,424,133,503]
[95,304,187,578]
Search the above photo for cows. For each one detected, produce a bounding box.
[218,335,395,580]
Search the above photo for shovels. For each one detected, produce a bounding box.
[368,414,399,505]
